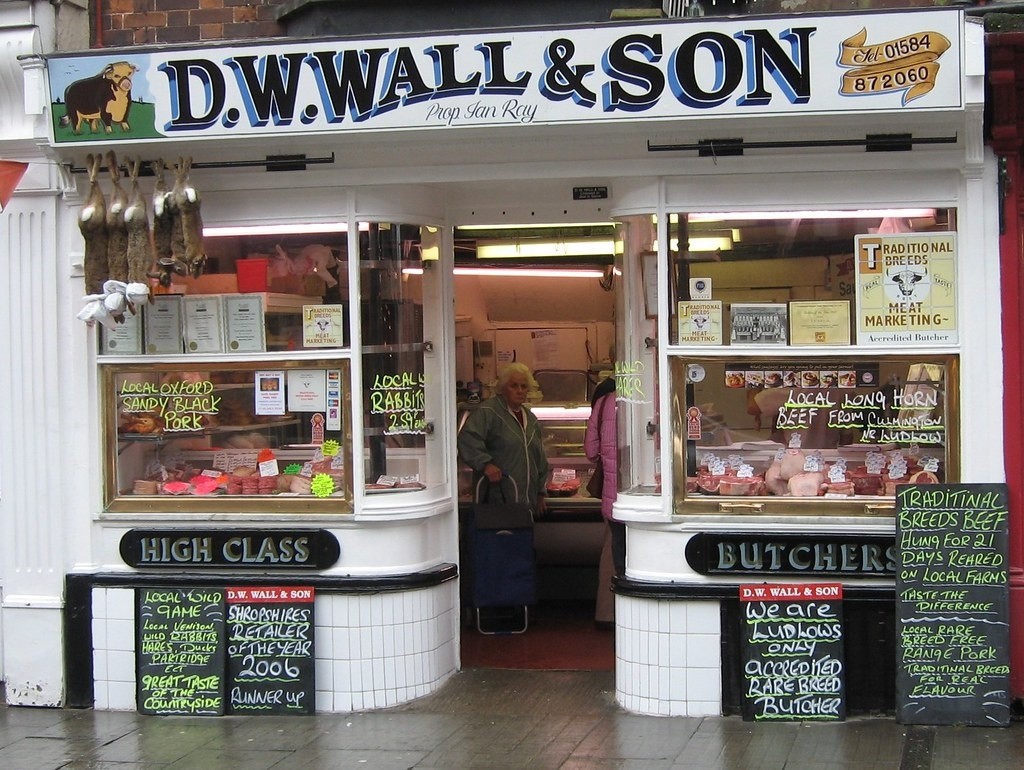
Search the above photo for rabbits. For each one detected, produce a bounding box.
[79,152,208,332]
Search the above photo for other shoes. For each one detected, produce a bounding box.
[594,621,615,632]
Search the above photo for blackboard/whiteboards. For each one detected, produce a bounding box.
[134,587,225,717]
[225,587,315,716]
[895,484,1009,727]
[496,328,587,379]
[739,583,844,723]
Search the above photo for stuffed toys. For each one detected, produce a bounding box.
[73,149,207,333]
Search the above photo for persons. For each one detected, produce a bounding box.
[457,363,550,628]
[584,371,625,632]
[746,384,856,449]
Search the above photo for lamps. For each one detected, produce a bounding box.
[474,231,737,259]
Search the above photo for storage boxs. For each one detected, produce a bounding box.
[236,258,273,293]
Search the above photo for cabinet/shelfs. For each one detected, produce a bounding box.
[156,289,322,453]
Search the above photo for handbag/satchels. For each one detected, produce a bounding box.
[586,457,604,499]
